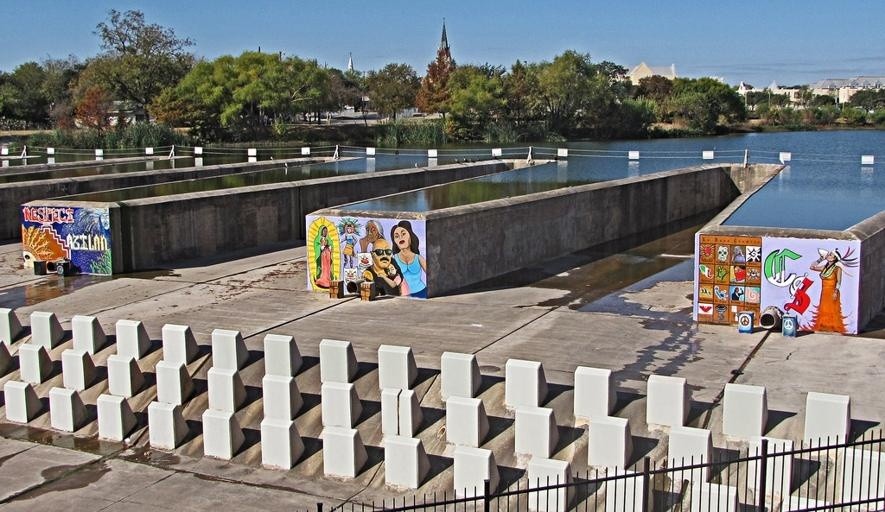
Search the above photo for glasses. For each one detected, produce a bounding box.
[371,248,394,257]
